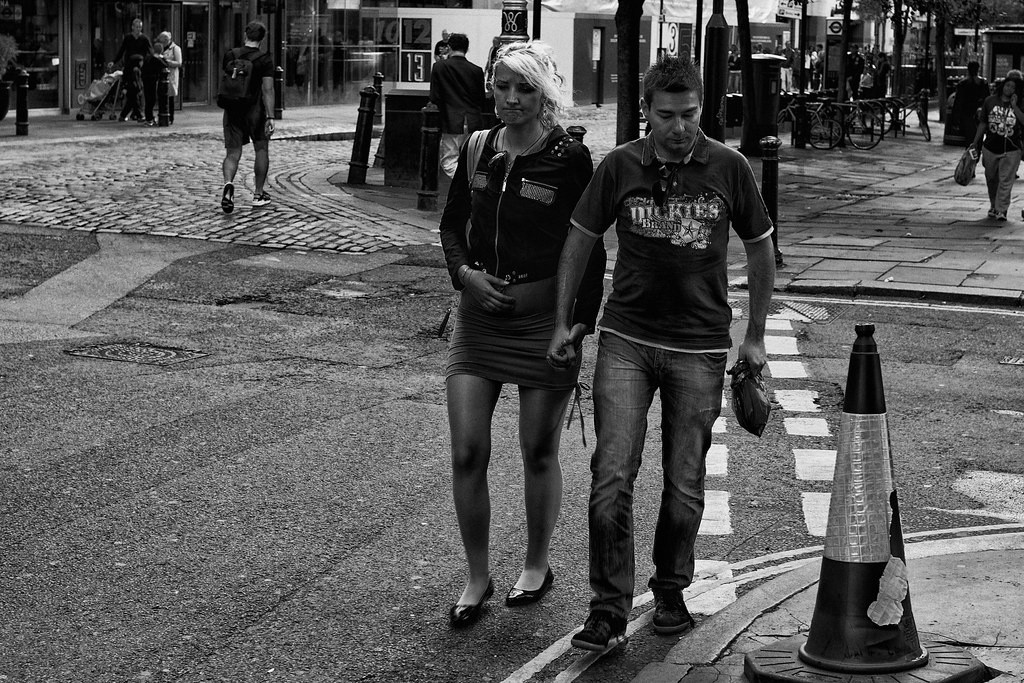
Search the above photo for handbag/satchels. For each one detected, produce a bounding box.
[725,361,770,438]
[954,146,979,187]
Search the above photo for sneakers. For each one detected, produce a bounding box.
[652,585,693,634]
[252,192,270,207]
[571,609,628,651]
[221,181,235,214]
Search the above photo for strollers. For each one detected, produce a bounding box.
[76,64,124,122]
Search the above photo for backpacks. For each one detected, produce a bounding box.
[216,48,266,110]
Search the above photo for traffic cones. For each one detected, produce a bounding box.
[744,324,994,683]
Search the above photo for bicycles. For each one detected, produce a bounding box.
[775,90,935,151]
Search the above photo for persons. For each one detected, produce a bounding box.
[93,38,103,67]
[950,61,1024,222]
[286,27,374,99]
[437,41,609,628]
[426,29,501,179]
[545,55,778,653]
[107,19,182,128]
[677,39,936,99]
[216,21,275,213]
[35,39,48,61]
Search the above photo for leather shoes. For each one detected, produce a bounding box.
[450,578,494,626]
[505,566,554,606]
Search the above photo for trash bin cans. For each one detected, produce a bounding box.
[385,89,431,188]
[944,76,968,146]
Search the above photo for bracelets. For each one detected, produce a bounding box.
[459,267,474,294]
[267,117,274,120]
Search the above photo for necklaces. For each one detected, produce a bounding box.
[500,118,545,172]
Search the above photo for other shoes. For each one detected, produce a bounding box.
[988,205,996,217]
[130,114,146,121]
[996,211,1007,221]
[143,119,155,126]
[119,116,128,121]
